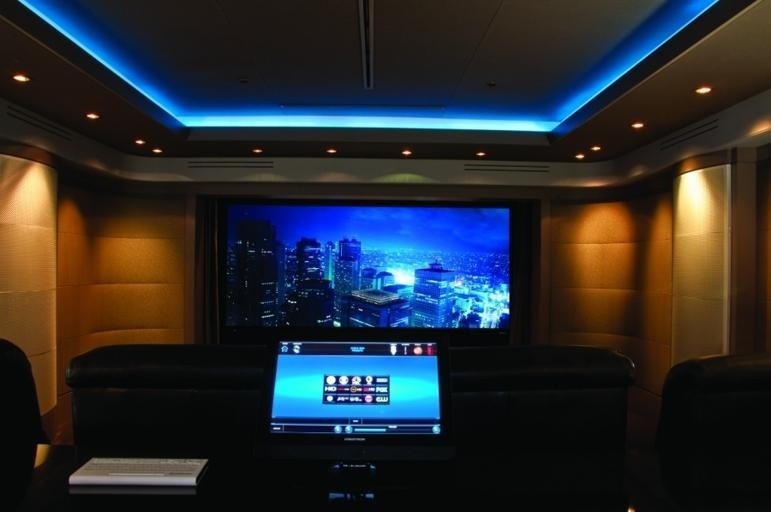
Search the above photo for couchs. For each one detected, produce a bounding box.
[64,342,635,498]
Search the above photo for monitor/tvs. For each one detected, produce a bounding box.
[257,341,453,492]
[222,203,512,346]
